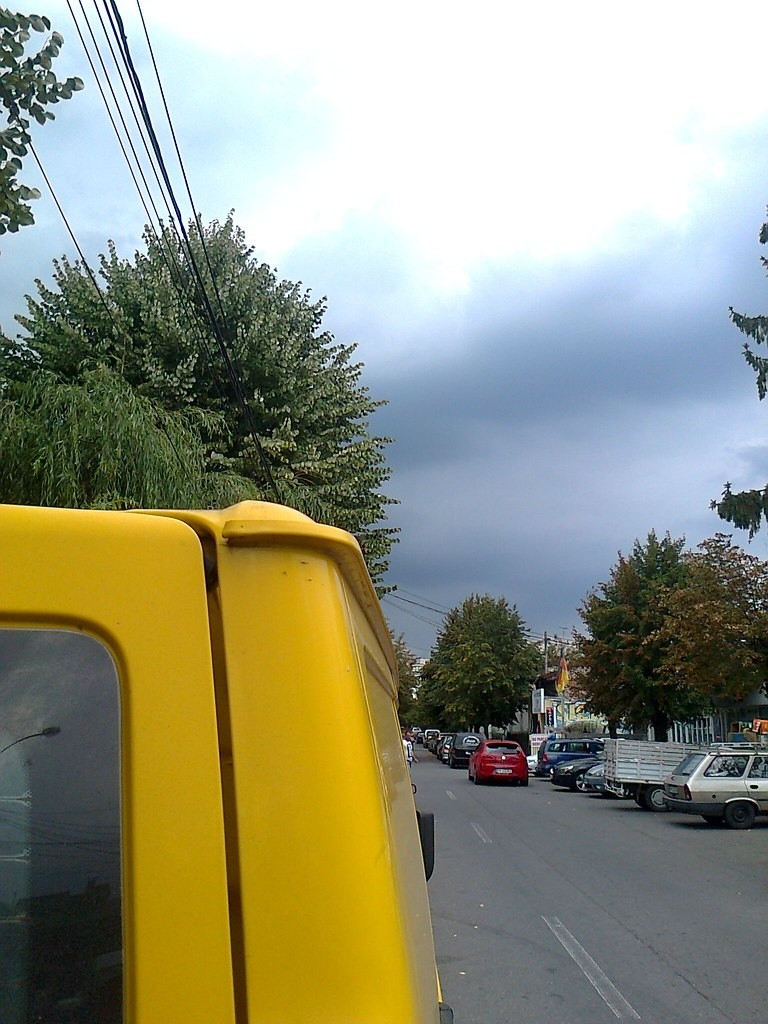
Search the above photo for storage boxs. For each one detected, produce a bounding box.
[727,720,768,746]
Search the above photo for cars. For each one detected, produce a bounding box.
[584,763,605,796]
[411,725,453,765]
[526,755,538,775]
[661,742,768,830]
[549,750,606,793]
[465,739,530,787]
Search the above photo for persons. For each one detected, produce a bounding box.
[583,727,588,733]
[547,728,556,739]
[402,731,418,783]
[715,736,722,742]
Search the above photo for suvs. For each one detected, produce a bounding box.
[446,733,489,768]
[535,738,607,777]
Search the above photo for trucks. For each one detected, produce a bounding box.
[1,497,456,1024]
[602,739,709,812]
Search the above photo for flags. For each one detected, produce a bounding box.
[555,649,570,693]
